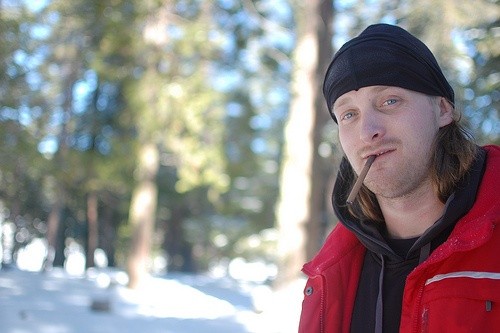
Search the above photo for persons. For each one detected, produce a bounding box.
[295,22,500,333]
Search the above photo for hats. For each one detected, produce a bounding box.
[322,23,455,125]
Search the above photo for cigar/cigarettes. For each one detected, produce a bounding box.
[345,154,377,206]
